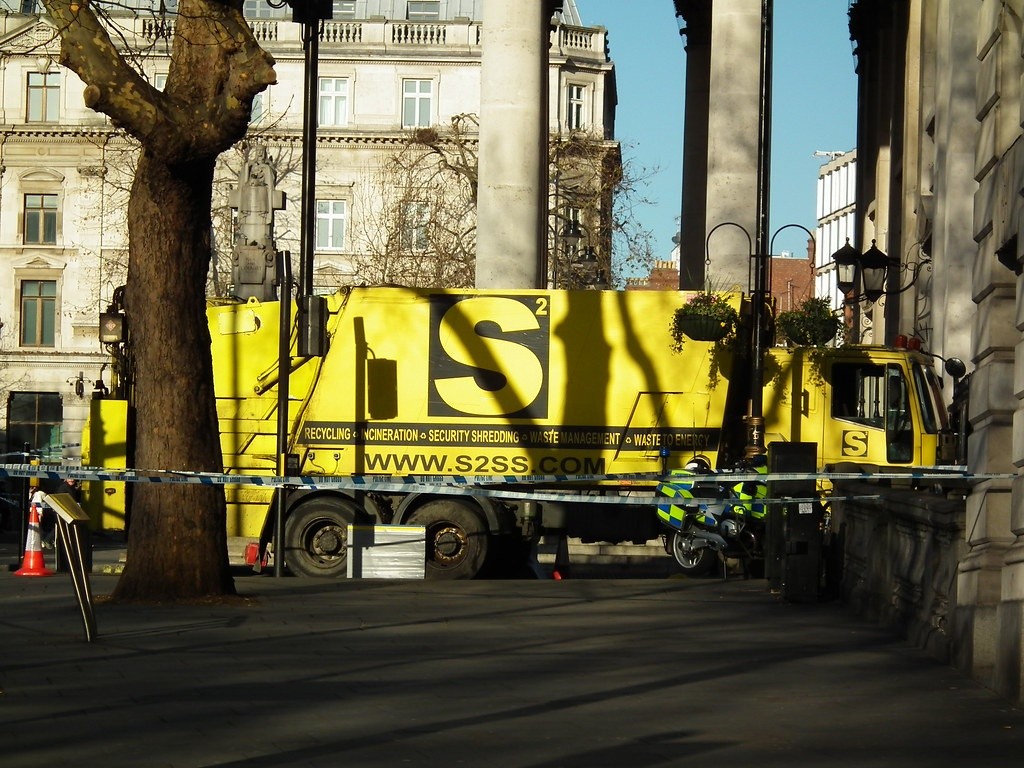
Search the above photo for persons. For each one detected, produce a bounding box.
[44,476,77,549]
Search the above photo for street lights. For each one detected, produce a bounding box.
[831,238,890,345]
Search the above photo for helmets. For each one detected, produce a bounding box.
[688,454,711,473]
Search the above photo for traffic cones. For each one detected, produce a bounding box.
[14,503,53,577]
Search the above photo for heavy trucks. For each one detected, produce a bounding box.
[66,284,966,579]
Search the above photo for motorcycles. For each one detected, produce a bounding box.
[654,454,832,574]
